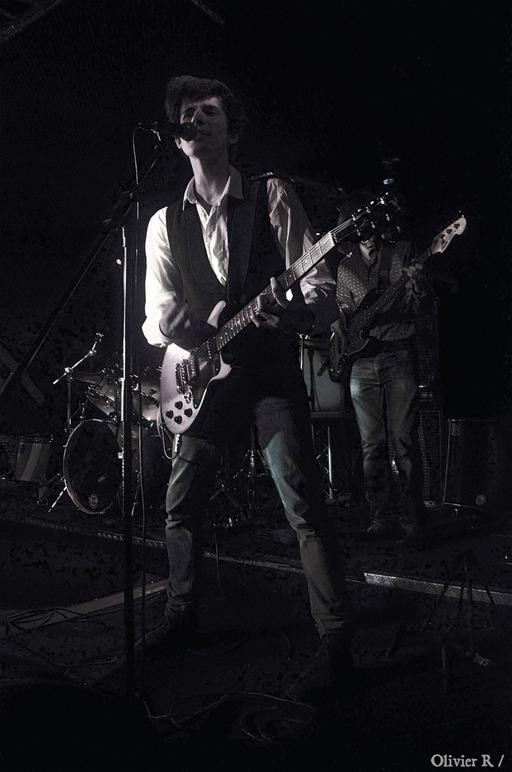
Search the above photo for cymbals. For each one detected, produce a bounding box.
[71,366,103,383]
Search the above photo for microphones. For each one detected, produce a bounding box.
[140,121,197,140]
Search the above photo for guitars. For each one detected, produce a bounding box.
[317,208,467,383]
[160,189,404,439]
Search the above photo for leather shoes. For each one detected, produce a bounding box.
[363,511,418,544]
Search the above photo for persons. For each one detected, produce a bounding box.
[330,190,434,544]
[133,75,355,701]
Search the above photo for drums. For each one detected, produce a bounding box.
[86,350,161,428]
[63,419,173,515]
[2,432,63,482]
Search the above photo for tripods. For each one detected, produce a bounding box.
[38,330,105,513]
[418,548,504,657]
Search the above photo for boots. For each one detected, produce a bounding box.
[135,597,190,658]
[256,640,355,742]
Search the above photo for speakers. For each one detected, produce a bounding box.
[364,291,444,514]
[442,413,511,519]
